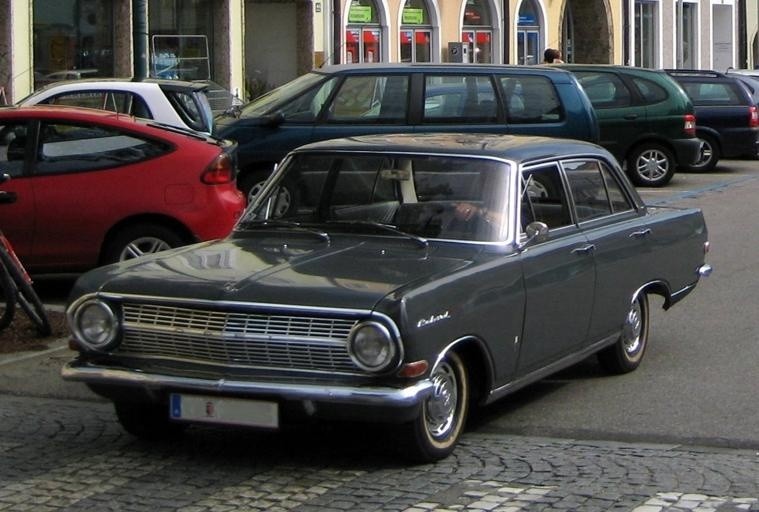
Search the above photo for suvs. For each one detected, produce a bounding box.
[536,62,702,186]
[658,67,759,173]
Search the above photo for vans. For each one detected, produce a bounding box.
[193,59,601,224]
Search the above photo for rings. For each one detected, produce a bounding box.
[466,208,470,213]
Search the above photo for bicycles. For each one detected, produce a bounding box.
[0,170,55,340]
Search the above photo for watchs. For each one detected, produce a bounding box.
[479,206,492,226]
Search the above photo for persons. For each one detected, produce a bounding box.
[452,171,523,237]
[542,47,568,64]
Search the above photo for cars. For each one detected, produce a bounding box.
[409,83,528,120]
[720,68,759,110]
[54,130,713,458]
[10,76,216,143]
[34,57,207,85]
[1,105,248,287]
